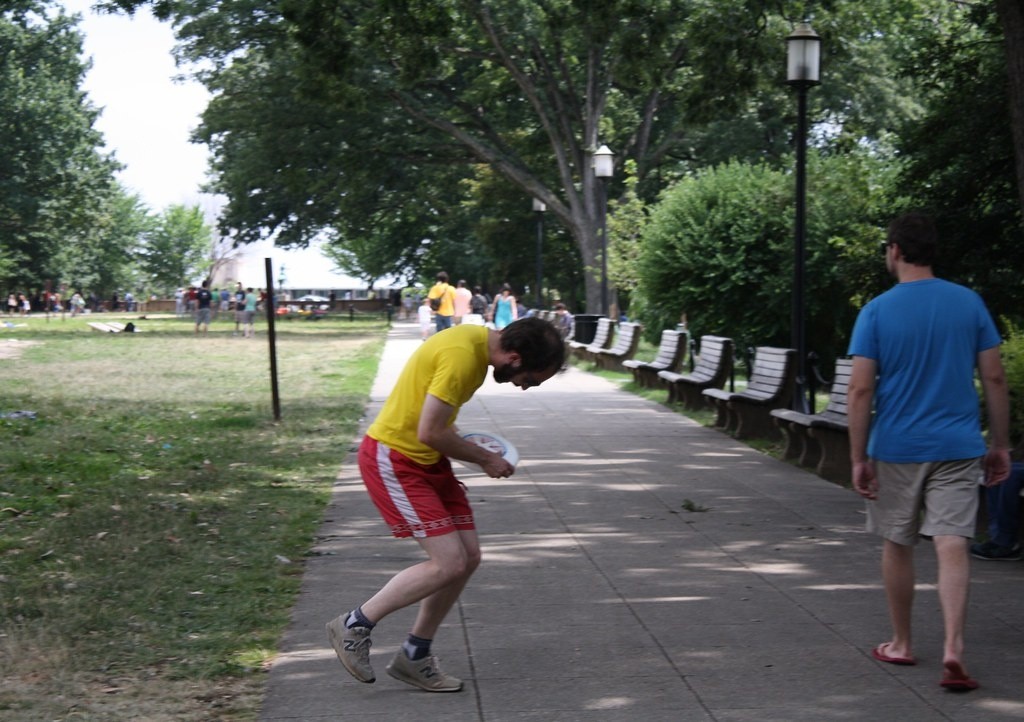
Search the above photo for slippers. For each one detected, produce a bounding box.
[939,675,979,690]
[871,642,914,663]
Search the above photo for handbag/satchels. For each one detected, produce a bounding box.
[429,289,446,311]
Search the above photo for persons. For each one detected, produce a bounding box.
[393,271,575,371]
[175,281,256,338]
[44,289,81,313]
[9,289,26,317]
[89,291,133,312]
[846,217,1011,690]
[327,316,565,691]
[261,290,335,320]
[344,286,376,300]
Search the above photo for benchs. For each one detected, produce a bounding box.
[569,317,615,367]
[562,314,577,360]
[538,308,548,321]
[774,357,864,477]
[518,307,538,319]
[621,327,688,386]
[588,321,643,371]
[658,336,736,410]
[701,346,810,443]
[547,311,559,325]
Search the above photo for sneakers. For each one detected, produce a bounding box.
[327,610,375,683]
[384,649,464,691]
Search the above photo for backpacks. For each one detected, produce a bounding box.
[472,295,485,315]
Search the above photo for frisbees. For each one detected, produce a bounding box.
[455,430,519,472]
